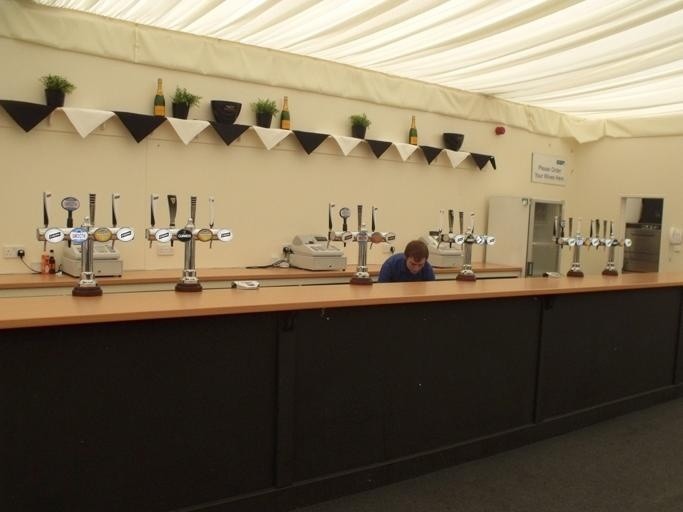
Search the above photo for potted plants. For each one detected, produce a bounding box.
[252,97,279,128]
[39,73,76,107]
[169,84,202,119]
[351,112,370,138]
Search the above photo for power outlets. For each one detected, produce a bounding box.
[3,245,24,259]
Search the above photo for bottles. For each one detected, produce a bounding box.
[41,248,55,273]
[408,115,418,146]
[280,96,291,131]
[153,78,166,118]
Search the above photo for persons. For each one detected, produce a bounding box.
[377,239,434,282]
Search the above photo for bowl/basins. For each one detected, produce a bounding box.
[210,100,241,124]
[443,132,464,152]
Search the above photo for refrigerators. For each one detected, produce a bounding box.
[485,193,567,277]
